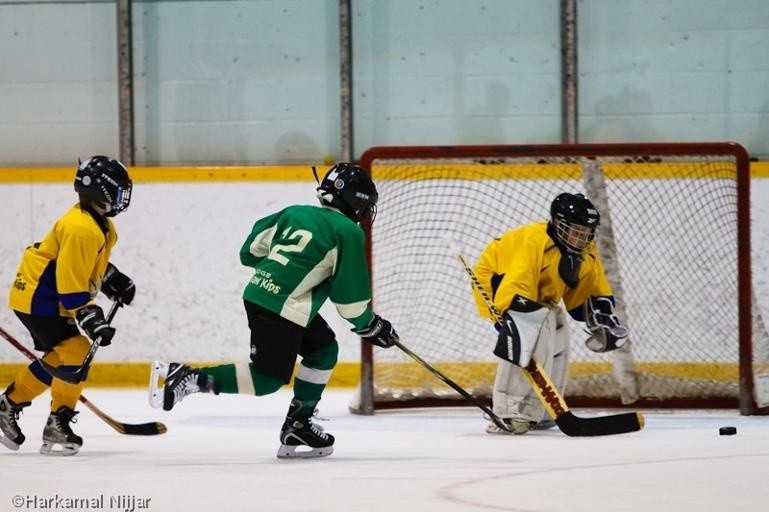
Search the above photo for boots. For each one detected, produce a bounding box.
[42,400,83,446]
[164,363,218,410]
[0,384,31,445]
[280,399,334,447]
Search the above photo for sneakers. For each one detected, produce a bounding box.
[531,421,557,432]
[487,416,531,434]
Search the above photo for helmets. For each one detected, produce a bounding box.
[551,193,599,257]
[316,162,378,229]
[75,155,132,216]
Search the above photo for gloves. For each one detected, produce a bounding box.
[100,262,135,307]
[76,305,115,346]
[351,314,398,348]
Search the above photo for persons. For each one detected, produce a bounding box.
[0,155,136,447]
[163,162,399,448]
[471,193,629,434]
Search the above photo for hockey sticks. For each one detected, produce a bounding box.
[0,328,167,436]
[40,298,122,383]
[394,340,533,433]
[443,231,644,437]
